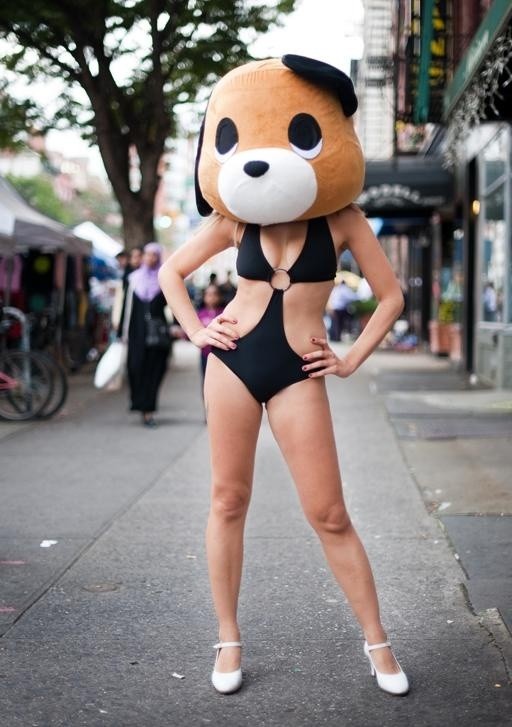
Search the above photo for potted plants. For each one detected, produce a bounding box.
[427,292,462,360]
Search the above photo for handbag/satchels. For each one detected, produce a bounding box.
[93,343,128,392]
[145,319,170,348]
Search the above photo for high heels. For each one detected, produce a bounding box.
[211,641,244,693]
[363,641,409,694]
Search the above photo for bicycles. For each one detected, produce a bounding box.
[0,304,69,424]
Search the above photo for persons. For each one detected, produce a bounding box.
[109,242,170,427]
[156,55,412,696]
[482,277,504,321]
[183,270,244,421]
[325,275,374,344]
